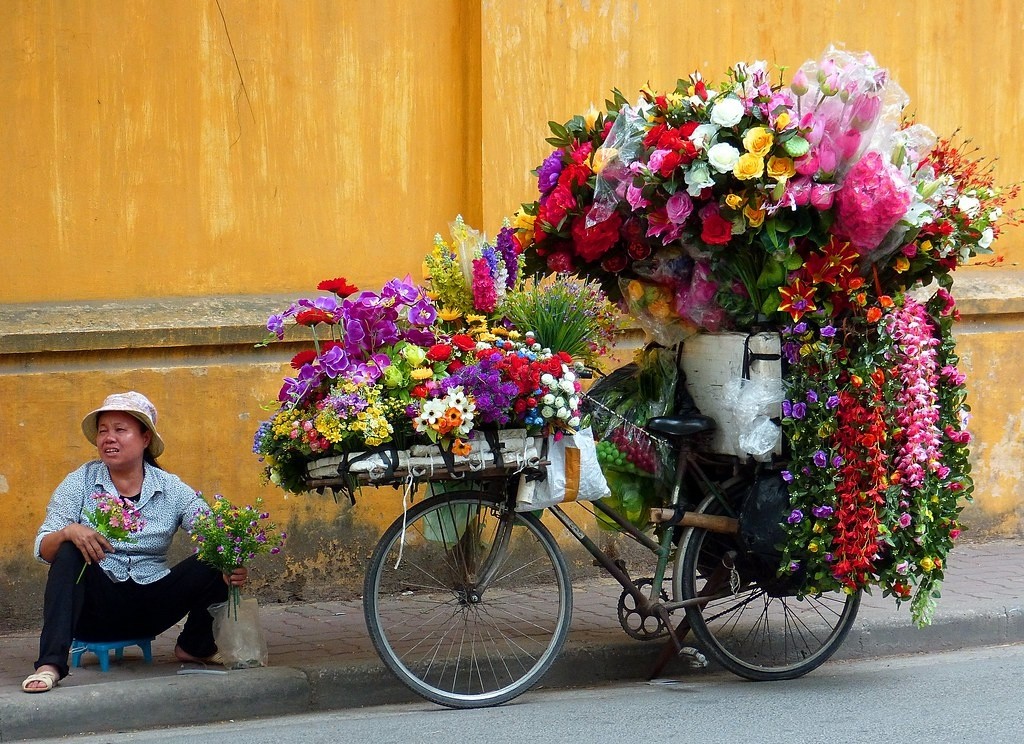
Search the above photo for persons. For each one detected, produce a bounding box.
[22,391,247,692]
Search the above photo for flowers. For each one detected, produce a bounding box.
[74,489,148,588]
[186,41,1024,628]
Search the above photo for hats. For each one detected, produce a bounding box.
[81,391,164,459]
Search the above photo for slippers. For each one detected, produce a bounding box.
[22,672,58,692]
[190,651,223,666]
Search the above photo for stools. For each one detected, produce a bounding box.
[72,636,157,673]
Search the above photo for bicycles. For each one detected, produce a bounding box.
[365,413,864,709]
